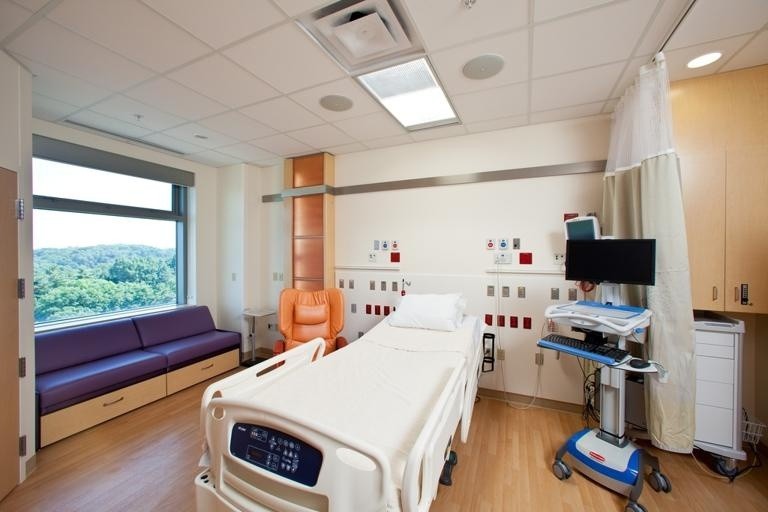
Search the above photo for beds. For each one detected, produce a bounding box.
[192,313,485,512]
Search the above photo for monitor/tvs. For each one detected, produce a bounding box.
[565,239,656,306]
[563,216,600,240]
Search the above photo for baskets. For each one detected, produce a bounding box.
[742,418,766,445]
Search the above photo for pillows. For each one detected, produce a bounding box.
[388,295,467,329]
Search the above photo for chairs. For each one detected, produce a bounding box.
[274,287,347,370]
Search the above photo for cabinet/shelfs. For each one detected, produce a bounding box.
[692,315,752,472]
[676,148,768,313]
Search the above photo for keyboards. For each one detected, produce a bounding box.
[540,333,631,364]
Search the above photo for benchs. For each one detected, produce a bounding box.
[35,306,242,451]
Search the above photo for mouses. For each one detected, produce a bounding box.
[630,358,650,368]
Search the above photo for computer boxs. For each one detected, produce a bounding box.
[594,368,647,430]
